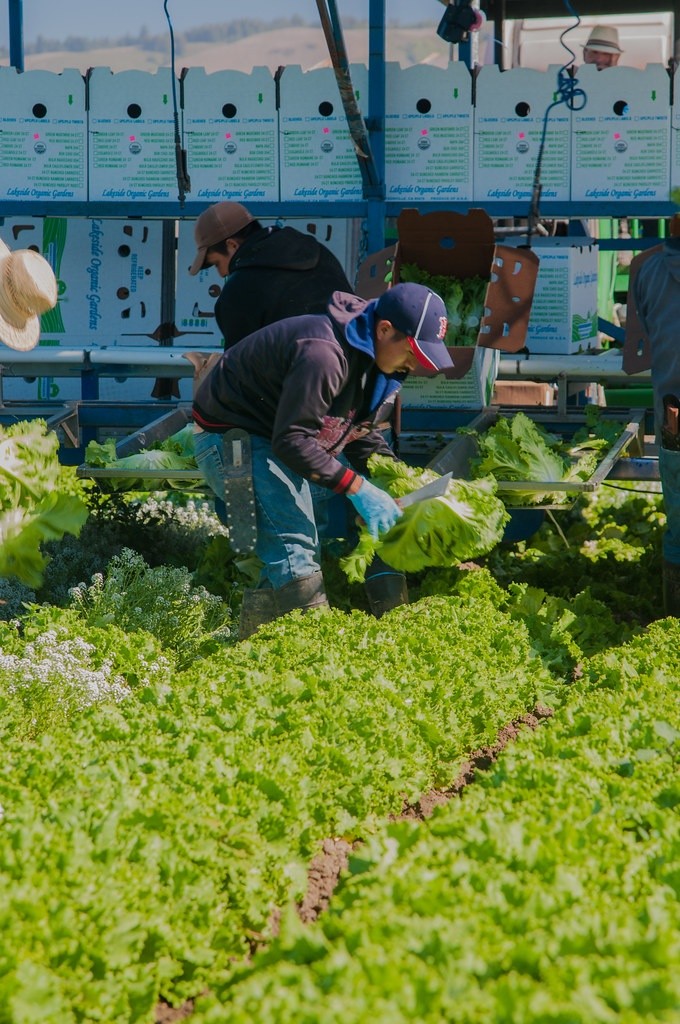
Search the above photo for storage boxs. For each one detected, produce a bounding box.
[0,64,679,201]
[0,215,601,355]
[1,260,607,407]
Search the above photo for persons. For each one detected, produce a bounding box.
[192,282,455,642]
[630,215,680,618]
[583,25,623,71]
[188,201,411,621]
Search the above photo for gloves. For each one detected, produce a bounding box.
[346,475,404,541]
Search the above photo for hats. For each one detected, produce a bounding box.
[0,239,58,351]
[189,201,255,276]
[376,281,455,371]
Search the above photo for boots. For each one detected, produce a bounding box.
[363,573,408,620]
[273,570,330,622]
[662,559,680,618]
[237,585,276,642]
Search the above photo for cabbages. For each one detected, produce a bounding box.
[0,256,680,1024]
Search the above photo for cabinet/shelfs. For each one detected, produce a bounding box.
[0,0,680,543]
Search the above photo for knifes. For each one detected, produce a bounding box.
[358,470,453,527]
